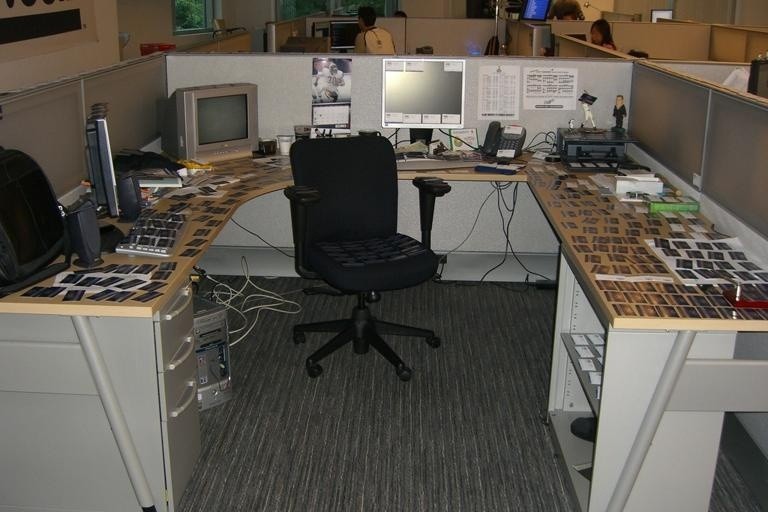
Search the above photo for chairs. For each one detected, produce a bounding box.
[284,137,450,382]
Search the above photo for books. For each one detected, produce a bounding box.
[647,195,699,212]
[137,174,183,188]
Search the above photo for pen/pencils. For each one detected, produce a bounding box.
[315,129,333,138]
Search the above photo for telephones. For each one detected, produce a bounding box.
[483,121,526,159]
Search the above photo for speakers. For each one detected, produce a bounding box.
[66,207,104,268]
[117,176,141,223]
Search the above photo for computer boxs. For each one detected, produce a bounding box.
[193,294,233,412]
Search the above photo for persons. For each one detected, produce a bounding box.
[314,128,333,136]
[392,10,407,18]
[355,6,396,54]
[314,63,346,102]
[548,0,584,20]
[612,95,627,129]
[579,89,596,130]
[590,18,616,51]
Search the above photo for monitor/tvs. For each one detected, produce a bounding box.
[651,9,673,23]
[382,59,466,154]
[521,0,552,22]
[161,83,259,165]
[60,118,125,255]
[330,20,361,53]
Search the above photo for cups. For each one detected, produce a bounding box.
[277,135,294,156]
[258,138,277,155]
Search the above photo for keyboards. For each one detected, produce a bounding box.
[115,212,191,258]
[395,152,480,170]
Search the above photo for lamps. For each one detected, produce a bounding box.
[583,1,603,14]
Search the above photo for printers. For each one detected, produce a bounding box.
[557,128,637,171]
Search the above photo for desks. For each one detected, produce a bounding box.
[0,151,768,511]
